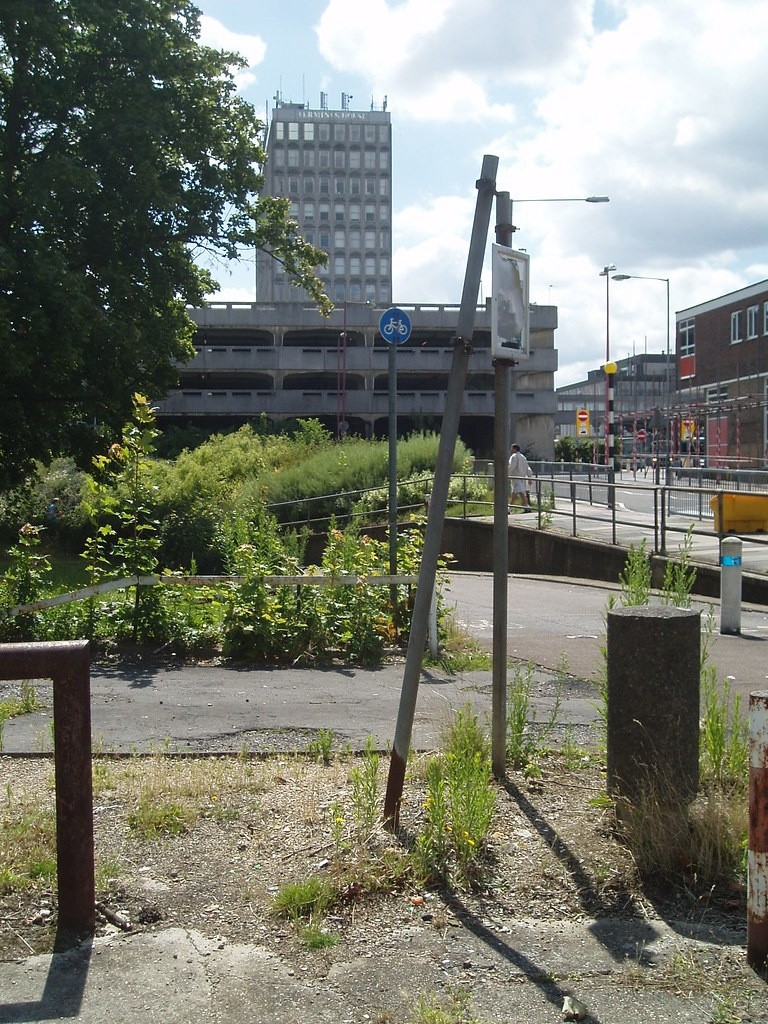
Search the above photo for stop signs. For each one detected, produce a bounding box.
[577,411,590,423]
[637,431,646,442]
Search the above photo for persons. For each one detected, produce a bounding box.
[508,443,534,514]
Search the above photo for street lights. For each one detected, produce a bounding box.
[492,191,610,781]
[599,263,619,365]
[602,362,618,508]
[612,274,671,481]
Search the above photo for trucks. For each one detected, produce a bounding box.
[652,439,675,469]
